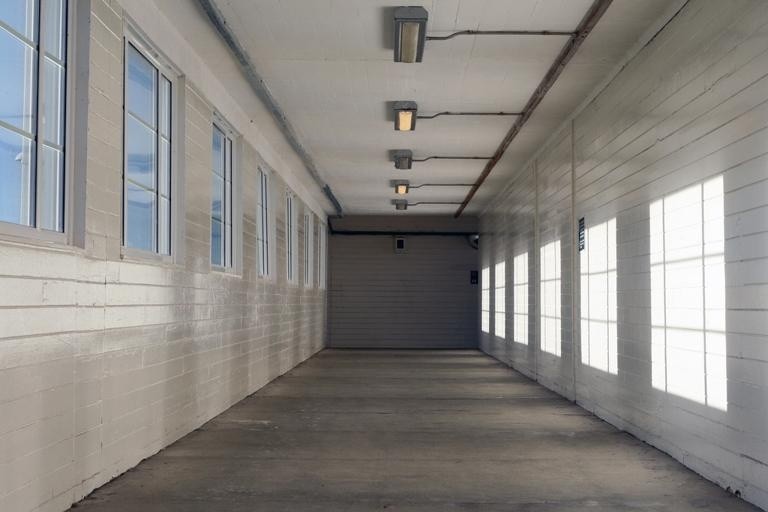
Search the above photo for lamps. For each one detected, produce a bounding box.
[394,101,417,132]
[395,199,408,212]
[394,180,409,195]
[393,7,426,64]
[392,150,413,170]
